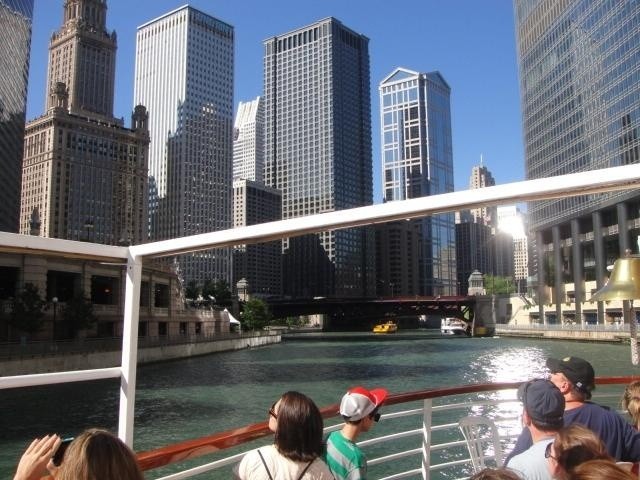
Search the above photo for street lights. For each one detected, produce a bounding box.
[84,220,94,240]
[51,297,58,342]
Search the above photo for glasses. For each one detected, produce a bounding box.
[368,413,380,422]
[269,400,277,419]
[544,441,556,461]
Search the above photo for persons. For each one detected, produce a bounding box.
[233,391,334,480]
[469,356,640,480]
[13,428,146,480]
[321,387,386,480]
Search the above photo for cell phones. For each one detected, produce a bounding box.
[52,436,74,468]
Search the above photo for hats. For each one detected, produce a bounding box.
[339,385,387,422]
[545,355,595,400]
[516,377,565,422]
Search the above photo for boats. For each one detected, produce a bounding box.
[373,320,398,333]
[441,317,488,336]
